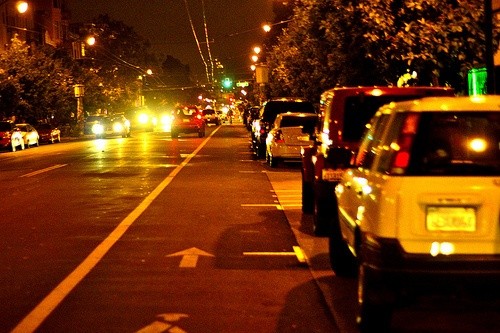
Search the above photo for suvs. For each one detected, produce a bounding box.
[300,85,457,239]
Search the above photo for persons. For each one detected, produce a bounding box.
[228,108,233,124]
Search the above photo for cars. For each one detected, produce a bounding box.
[0,120,61,152]
[328,94,500,333]
[244,105,260,130]
[171,106,228,139]
[264,112,319,167]
[83,113,131,139]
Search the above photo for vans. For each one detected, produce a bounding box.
[250,97,317,159]
